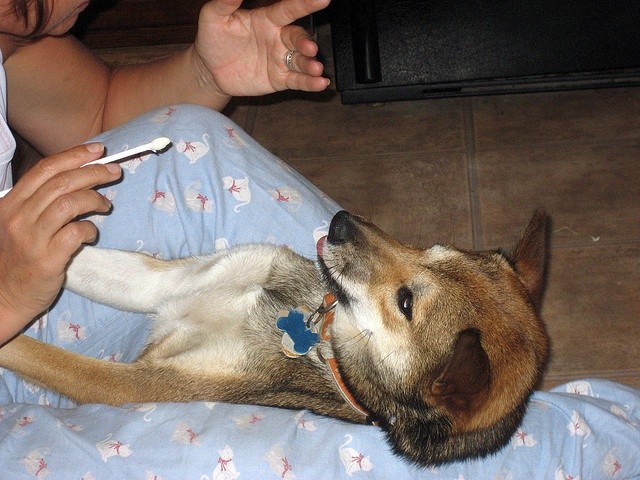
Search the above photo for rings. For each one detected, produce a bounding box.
[284,49,296,71]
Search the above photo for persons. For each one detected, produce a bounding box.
[0,0,330,347]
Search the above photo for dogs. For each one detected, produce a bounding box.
[0,190,556,471]
